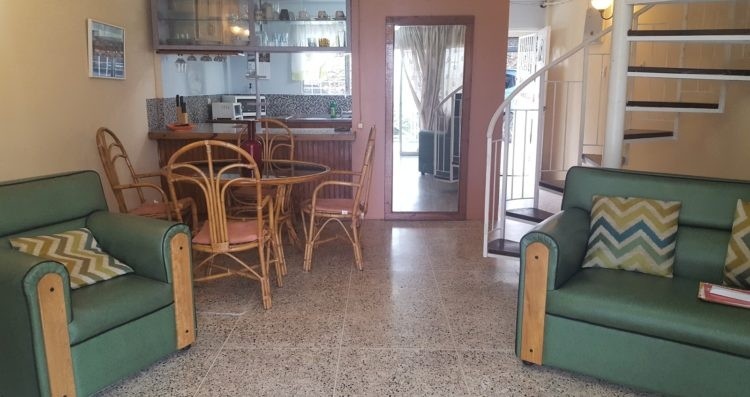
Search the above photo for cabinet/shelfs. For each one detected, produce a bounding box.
[151,0,347,51]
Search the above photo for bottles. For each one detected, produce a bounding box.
[330,101,336,118]
[242,122,262,177]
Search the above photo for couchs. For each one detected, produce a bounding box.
[0,169,197,397]
[514,166,750,397]
[419,118,459,174]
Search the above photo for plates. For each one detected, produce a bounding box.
[332,18,346,20]
[314,18,329,20]
[296,18,309,20]
[198,41,220,45]
[167,39,191,44]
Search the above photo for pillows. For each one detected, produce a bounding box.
[9,227,135,290]
[722,198,750,290]
[582,195,680,279]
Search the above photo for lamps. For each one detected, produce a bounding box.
[591,0,613,20]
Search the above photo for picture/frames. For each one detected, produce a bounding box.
[88,18,127,80]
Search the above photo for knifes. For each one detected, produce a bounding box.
[176,94,186,113]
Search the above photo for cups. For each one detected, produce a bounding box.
[308,38,316,48]
[318,11,325,18]
[271,32,280,46]
[280,33,288,46]
[338,32,345,47]
[255,11,262,21]
[336,11,343,17]
[319,38,326,47]
[299,11,306,18]
[255,31,267,46]
[261,6,272,19]
[281,9,288,20]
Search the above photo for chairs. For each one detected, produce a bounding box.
[301,126,375,272]
[166,140,282,310]
[232,118,294,247]
[96,127,198,231]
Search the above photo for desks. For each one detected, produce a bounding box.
[159,159,331,277]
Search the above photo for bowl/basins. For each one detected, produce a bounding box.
[231,39,249,46]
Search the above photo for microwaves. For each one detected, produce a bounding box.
[222,95,266,116]
[212,102,242,120]
